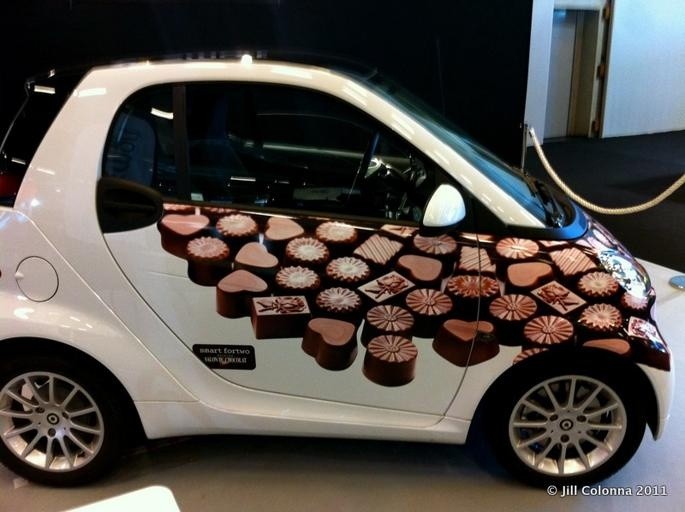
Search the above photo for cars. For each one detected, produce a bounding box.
[1,55,673,489]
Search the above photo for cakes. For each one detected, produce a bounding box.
[156,202,670,386]
